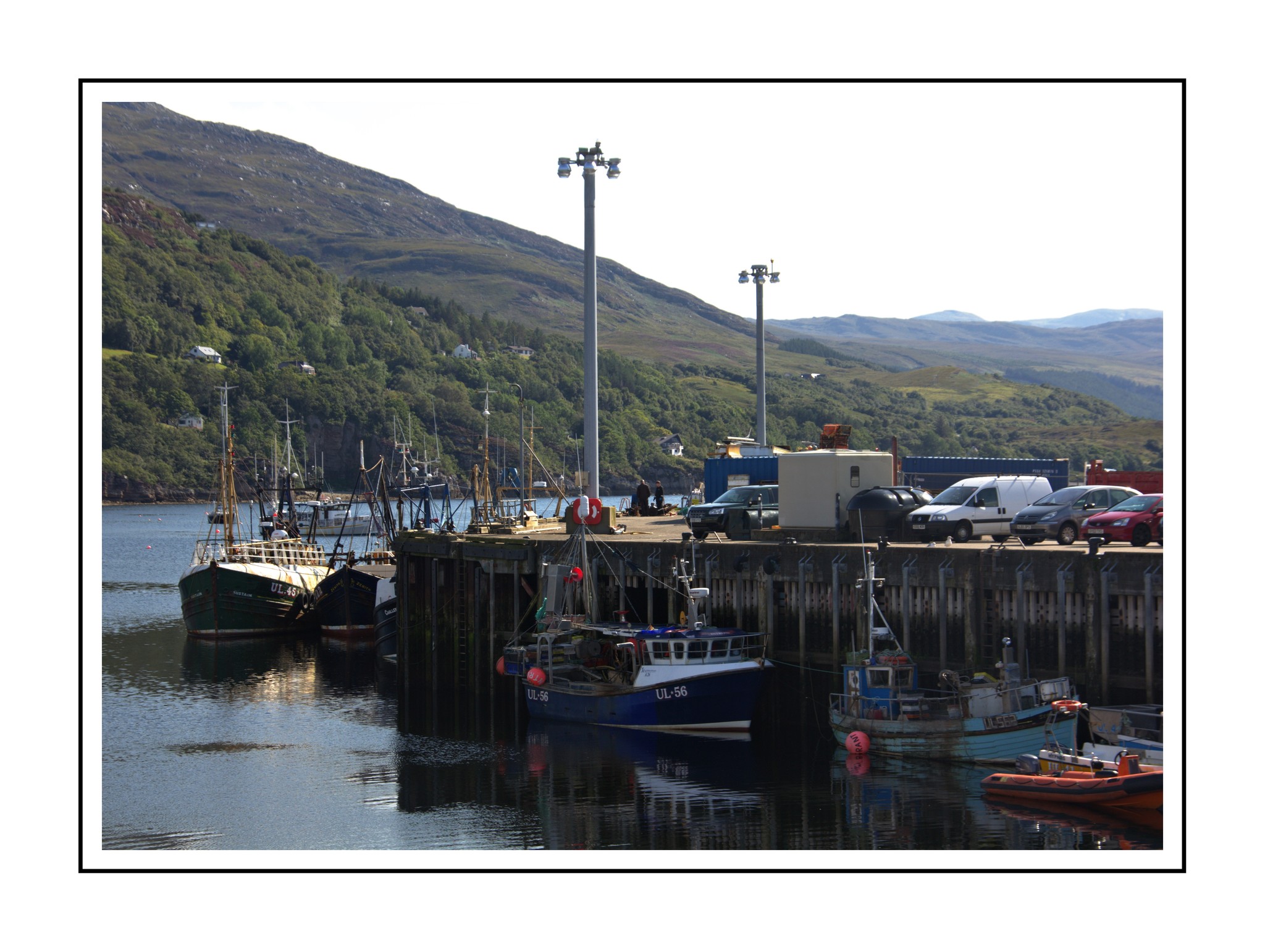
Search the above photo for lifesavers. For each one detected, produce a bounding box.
[879,656,908,661]
[665,628,691,633]
[879,661,908,664]
[1051,700,1082,711]
[299,592,308,609]
[577,502,597,518]
[307,592,314,608]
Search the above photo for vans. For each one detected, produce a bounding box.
[906,474,1059,544]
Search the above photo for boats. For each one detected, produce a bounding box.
[979,752,1163,819]
[1037,702,1163,775]
[176,380,549,663]
[496,431,779,743]
[828,503,1087,768]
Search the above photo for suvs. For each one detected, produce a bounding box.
[684,484,779,541]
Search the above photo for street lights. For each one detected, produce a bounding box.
[737,264,781,446]
[556,140,622,499]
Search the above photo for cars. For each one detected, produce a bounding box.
[1081,493,1163,548]
[1009,484,1147,546]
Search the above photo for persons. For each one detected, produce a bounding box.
[653,481,664,514]
[636,480,651,517]
